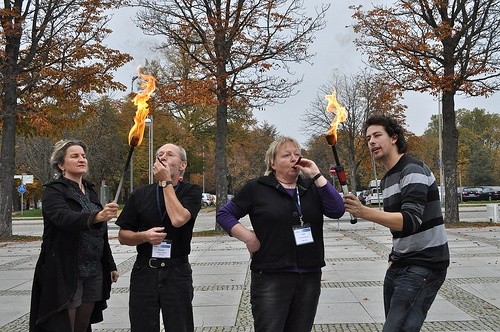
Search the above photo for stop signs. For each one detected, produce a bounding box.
[330,167,336,175]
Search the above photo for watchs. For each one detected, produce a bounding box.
[161,180,172,188]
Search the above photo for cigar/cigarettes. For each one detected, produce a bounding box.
[293,156,303,168]
[156,156,166,168]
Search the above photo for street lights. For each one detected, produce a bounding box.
[145,114,154,185]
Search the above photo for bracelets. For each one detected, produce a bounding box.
[312,173,322,182]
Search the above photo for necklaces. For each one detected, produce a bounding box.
[277,180,296,187]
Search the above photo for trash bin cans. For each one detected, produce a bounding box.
[487,204,496,219]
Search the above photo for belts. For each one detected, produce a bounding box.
[138,258,183,268]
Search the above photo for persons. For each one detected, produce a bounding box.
[115,144,201,332]
[217,137,344,332]
[29,139,119,332]
[343,116,450,332]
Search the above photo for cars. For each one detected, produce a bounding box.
[201,192,234,206]
[438,185,500,202]
[338,179,383,205]
